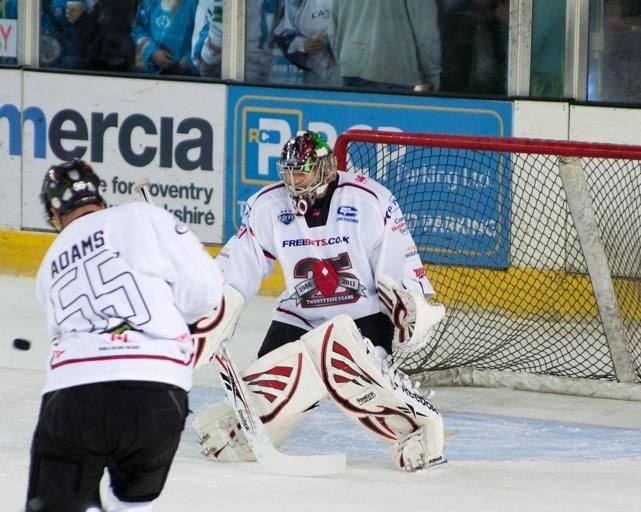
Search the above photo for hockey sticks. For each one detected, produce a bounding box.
[131,179,347,478]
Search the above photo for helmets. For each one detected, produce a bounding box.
[276,130,337,215]
[42,157,102,232]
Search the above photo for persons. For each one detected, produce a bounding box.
[38,0,513,99]
[187,129,446,473]
[24,157,225,512]
